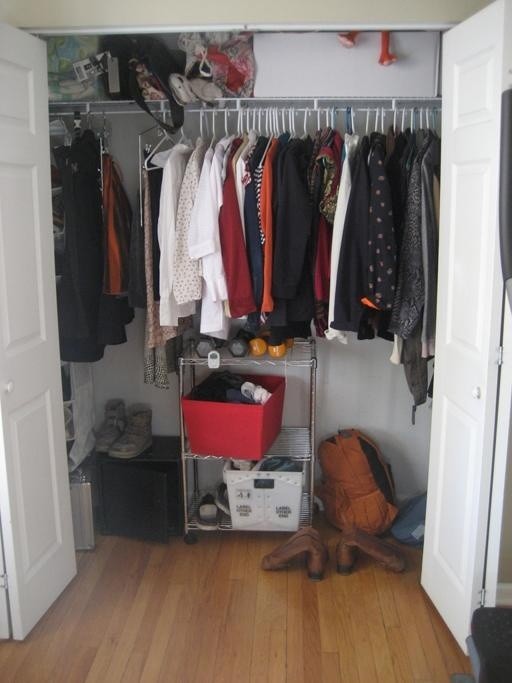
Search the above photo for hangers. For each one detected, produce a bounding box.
[48,106,111,155]
[144,106,440,172]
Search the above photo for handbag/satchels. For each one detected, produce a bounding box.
[97,32,260,135]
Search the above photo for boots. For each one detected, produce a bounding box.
[92,398,127,452]
[107,408,155,459]
[260,526,327,581]
[338,531,404,578]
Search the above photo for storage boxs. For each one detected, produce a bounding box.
[224,460,306,531]
[183,374,285,460]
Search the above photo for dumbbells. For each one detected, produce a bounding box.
[229,329,294,357]
[196,335,223,357]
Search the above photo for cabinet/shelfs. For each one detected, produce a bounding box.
[177,338,317,544]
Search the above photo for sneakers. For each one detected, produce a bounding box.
[196,492,219,530]
[215,483,230,515]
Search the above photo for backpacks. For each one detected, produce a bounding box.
[318,429,395,534]
